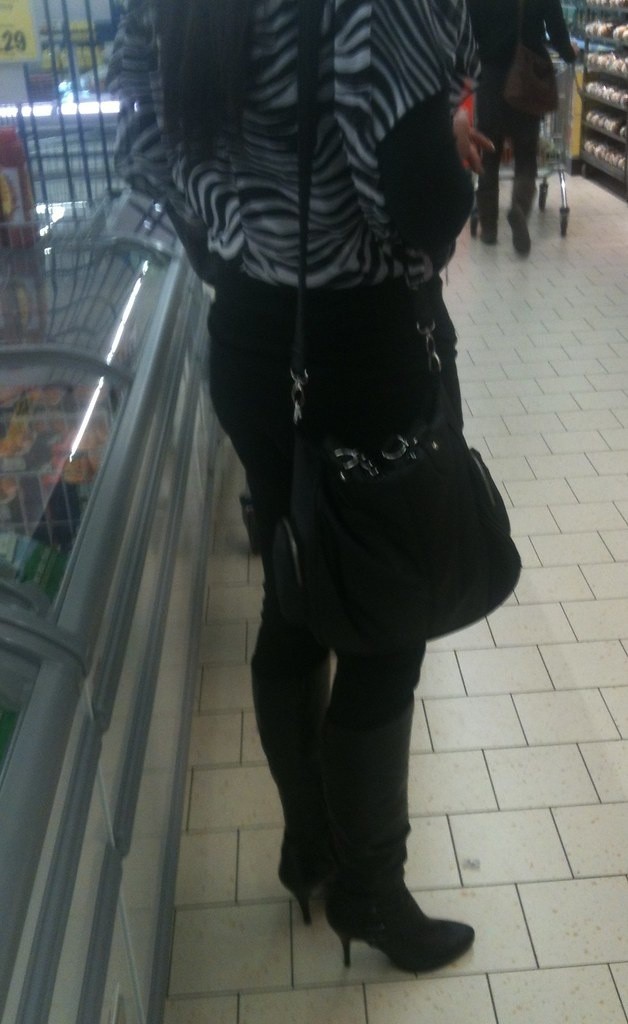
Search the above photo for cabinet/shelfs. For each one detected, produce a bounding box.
[570,0,628,187]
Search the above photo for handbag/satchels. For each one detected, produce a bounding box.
[504,40,558,118]
[271,417,521,655]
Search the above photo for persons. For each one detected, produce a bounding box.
[108,0,497,972]
[473,0,580,257]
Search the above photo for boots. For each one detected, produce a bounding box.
[504,175,536,255]
[475,184,500,244]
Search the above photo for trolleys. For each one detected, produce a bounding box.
[468,52,581,240]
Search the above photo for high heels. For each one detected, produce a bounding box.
[277,849,347,923]
[324,868,474,973]
